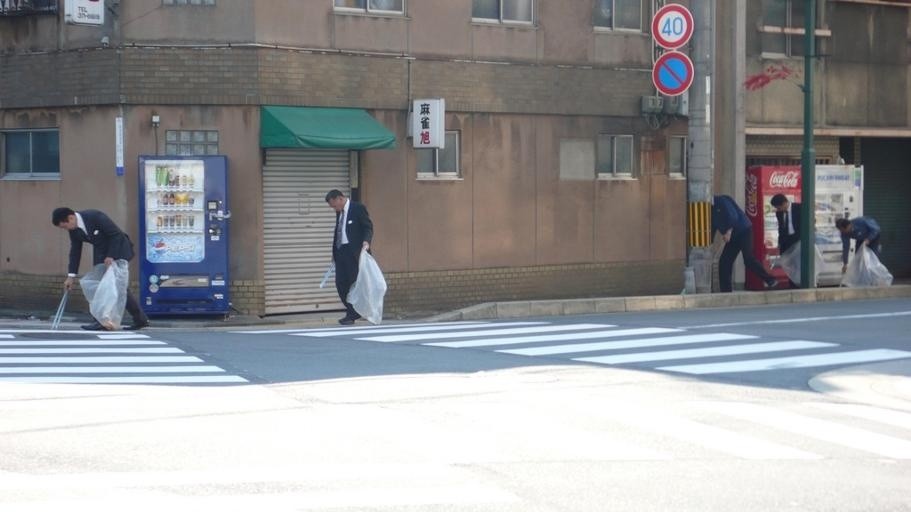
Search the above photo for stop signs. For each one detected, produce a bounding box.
[651,50,695,97]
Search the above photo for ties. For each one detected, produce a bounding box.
[335,212,344,250]
[784,210,790,237]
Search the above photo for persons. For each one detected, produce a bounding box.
[325,188,374,325]
[771,192,803,289]
[52,206,151,333]
[710,194,780,293]
[836,216,883,288]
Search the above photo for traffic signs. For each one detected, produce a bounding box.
[650,3,695,50]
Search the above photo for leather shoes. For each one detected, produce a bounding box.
[81,322,108,330]
[338,312,361,325]
[123,320,150,330]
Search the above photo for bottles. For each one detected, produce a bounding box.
[155,167,195,230]
